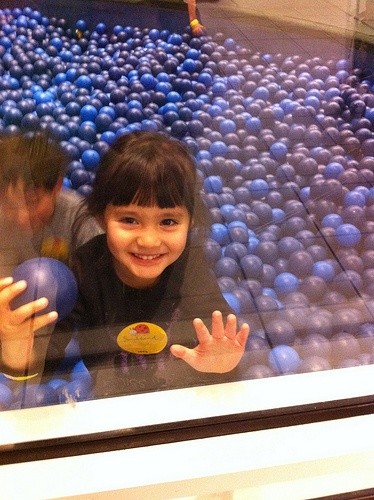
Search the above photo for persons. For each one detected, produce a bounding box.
[0,132,105,278]
[0,132,250,399]
[186,0,209,36]
